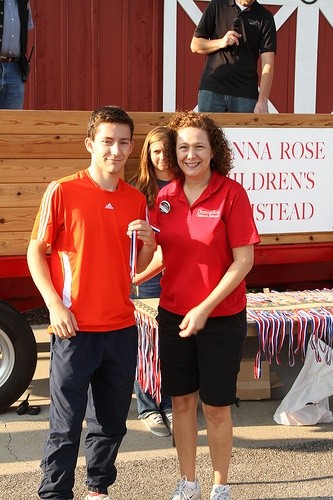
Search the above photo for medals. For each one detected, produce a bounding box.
[129,282,137,296]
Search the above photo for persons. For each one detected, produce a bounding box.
[124,126,176,436]
[190,0,277,113]
[127,113,261,500]
[27,106,156,500]
[0,0,34,109]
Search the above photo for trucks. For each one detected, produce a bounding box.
[0,1,333,414]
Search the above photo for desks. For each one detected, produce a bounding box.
[129,288,333,447]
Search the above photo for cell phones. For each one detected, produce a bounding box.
[28,406,40,415]
[16,393,30,415]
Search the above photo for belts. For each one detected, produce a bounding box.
[0,55,20,63]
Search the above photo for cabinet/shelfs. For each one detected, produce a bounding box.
[236,358,283,400]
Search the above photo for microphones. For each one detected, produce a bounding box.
[232,18,241,56]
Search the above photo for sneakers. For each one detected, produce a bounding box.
[85,491,111,500]
[210,485,231,500]
[168,475,201,500]
[163,408,173,434]
[141,412,169,436]
